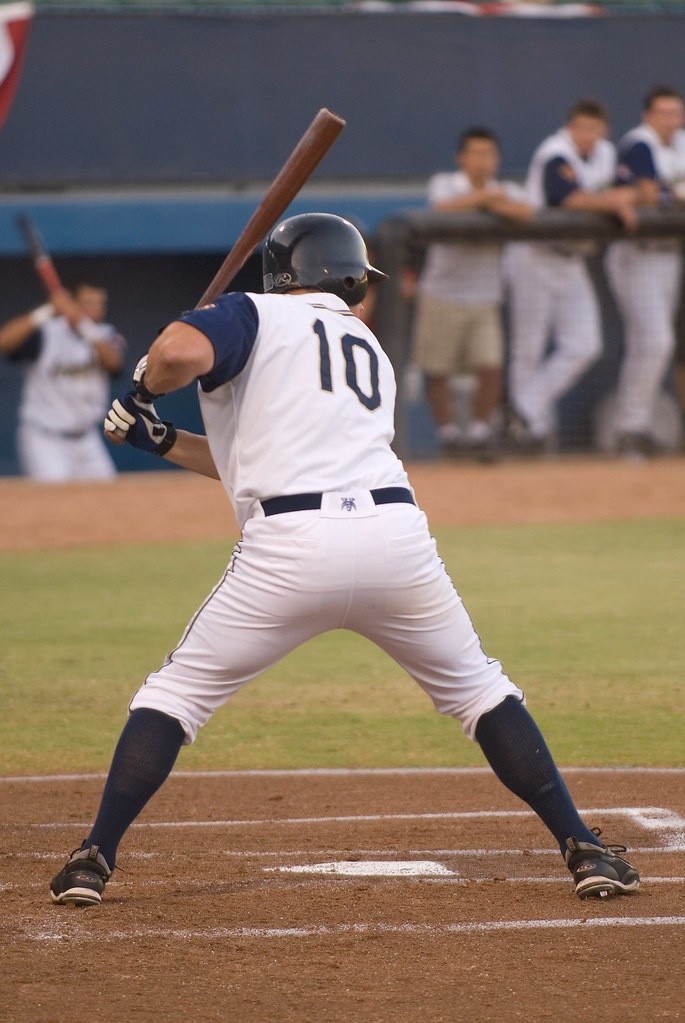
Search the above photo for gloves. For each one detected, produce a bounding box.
[105,355,177,457]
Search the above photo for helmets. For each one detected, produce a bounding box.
[261,210,390,298]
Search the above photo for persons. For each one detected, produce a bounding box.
[505,101,657,463]
[0,275,129,481]
[44,213,646,905]
[606,86,685,452]
[411,126,534,459]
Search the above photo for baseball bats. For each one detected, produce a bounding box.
[13,206,69,314]
[103,104,353,446]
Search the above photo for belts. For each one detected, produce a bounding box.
[261,487,417,517]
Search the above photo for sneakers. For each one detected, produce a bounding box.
[566,840,641,900]
[50,844,110,905]
